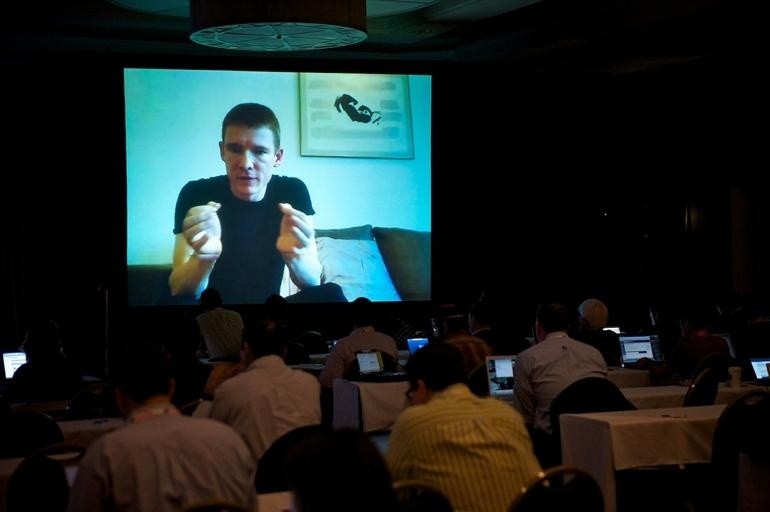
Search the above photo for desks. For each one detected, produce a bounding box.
[1,349,768,512]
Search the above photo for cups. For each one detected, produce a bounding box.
[728,366,743,388]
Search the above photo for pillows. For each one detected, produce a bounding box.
[282,219,402,304]
[373,226,431,296]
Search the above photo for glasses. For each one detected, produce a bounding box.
[405,384,420,400]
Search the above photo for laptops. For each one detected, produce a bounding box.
[603,327,620,333]
[2,351,28,379]
[407,336,428,355]
[617,334,663,364]
[749,357,770,382]
[485,354,518,403]
[357,350,388,375]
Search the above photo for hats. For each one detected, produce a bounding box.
[577,299,609,330]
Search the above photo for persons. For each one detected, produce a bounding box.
[169,102,348,305]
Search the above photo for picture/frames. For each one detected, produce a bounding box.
[295,72,416,160]
